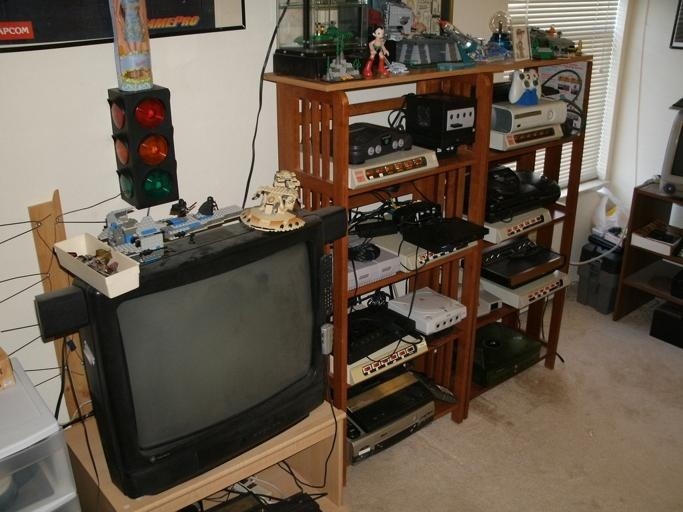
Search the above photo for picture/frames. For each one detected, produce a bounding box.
[669,2,681,49]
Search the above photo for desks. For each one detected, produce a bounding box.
[61,401,348,512]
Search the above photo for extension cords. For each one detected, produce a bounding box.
[237,478,271,503]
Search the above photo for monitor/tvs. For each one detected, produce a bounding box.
[73,208,325,500]
[657,110,683,198]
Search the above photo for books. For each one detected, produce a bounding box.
[588,223,683,268]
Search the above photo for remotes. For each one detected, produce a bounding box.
[317,254,335,326]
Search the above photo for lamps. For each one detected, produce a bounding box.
[107,83,178,208]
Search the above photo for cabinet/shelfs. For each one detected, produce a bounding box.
[262,55,594,486]
[614,189,682,318]
[0,357,83,511]
